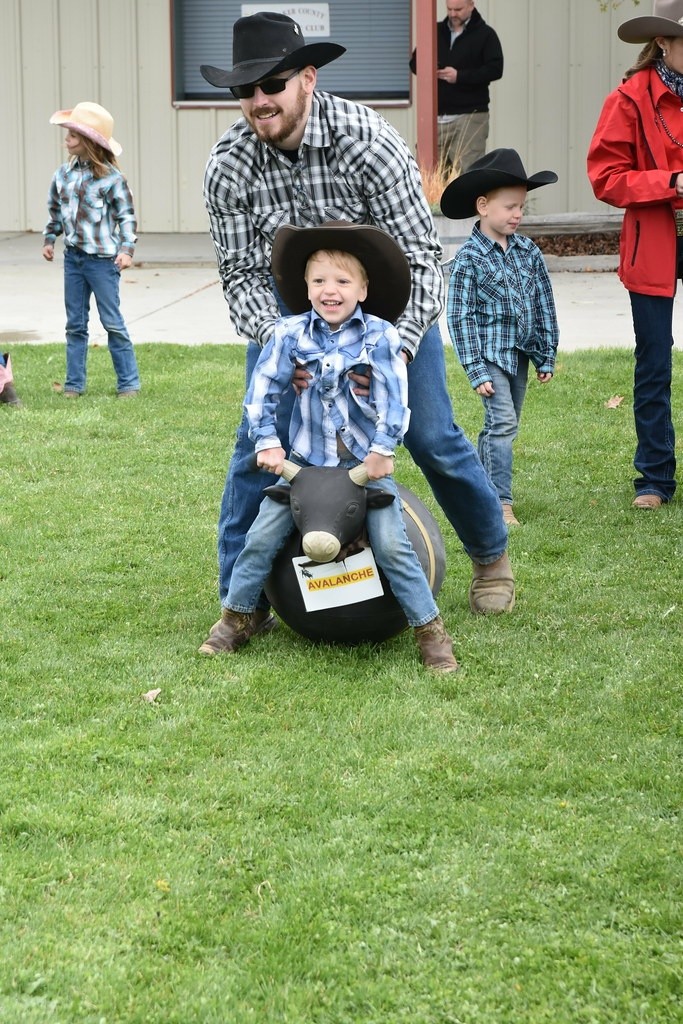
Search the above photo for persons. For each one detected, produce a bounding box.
[439,149,559,524]
[42,102,141,398]
[409,0,504,173]
[197,221,458,676]
[587,0,683,511]
[200,12,516,637]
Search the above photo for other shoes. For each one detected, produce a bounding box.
[117,390,138,399]
[502,504,520,525]
[64,391,79,399]
[632,495,663,510]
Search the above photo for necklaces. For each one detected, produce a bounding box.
[657,106,683,148]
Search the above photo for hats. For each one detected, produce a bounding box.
[617,0,683,44]
[49,102,122,156]
[271,220,411,323]
[200,12,347,88]
[440,148,559,220]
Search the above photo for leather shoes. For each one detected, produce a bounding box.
[469,549,515,616]
[209,608,275,638]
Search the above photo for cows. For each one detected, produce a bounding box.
[264,451,447,646]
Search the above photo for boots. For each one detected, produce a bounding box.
[199,608,253,657]
[413,615,458,674]
[0,353,21,404]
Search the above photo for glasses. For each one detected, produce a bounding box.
[230,66,305,98]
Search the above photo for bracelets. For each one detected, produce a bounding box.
[402,347,413,364]
